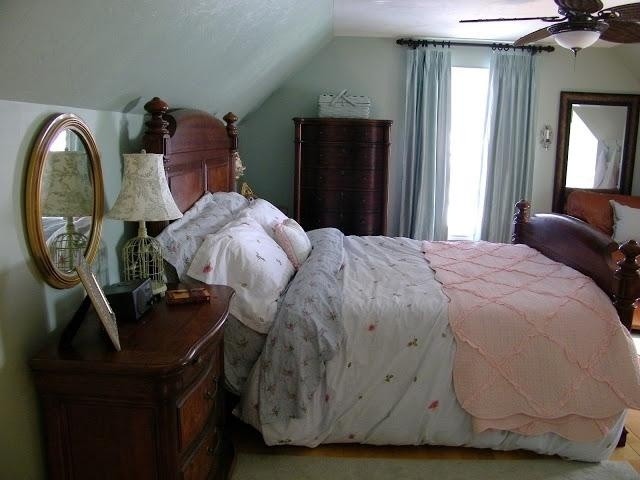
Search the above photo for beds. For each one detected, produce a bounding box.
[134,88,640,467]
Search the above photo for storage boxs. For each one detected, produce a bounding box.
[315,91,371,123]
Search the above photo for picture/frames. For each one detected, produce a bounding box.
[59,248,124,356]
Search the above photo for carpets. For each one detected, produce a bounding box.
[623,398,640,442]
[227,448,640,480]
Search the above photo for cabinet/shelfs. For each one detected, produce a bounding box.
[289,113,393,240]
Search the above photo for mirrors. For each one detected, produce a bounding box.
[18,107,108,295]
[551,90,640,219]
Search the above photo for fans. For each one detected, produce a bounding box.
[457,0,639,51]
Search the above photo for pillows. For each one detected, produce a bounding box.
[274,217,313,270]
[151,191,233,286]
[187,216,296,337]
[209,188,249,218]
[605,198,640,248]
[239,196,290,241]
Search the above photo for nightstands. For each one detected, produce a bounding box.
[27,271,245,478]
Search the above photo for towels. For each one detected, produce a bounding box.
[592,149,606,190]
[604,147,620,191]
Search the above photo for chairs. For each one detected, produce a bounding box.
[564,185,640,334]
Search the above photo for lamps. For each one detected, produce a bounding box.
[40,139,96,278]
[107,144,185,307]
[548,17,609,60]
[539,125,551,150]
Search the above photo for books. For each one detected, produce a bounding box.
[165,287,211,307]
